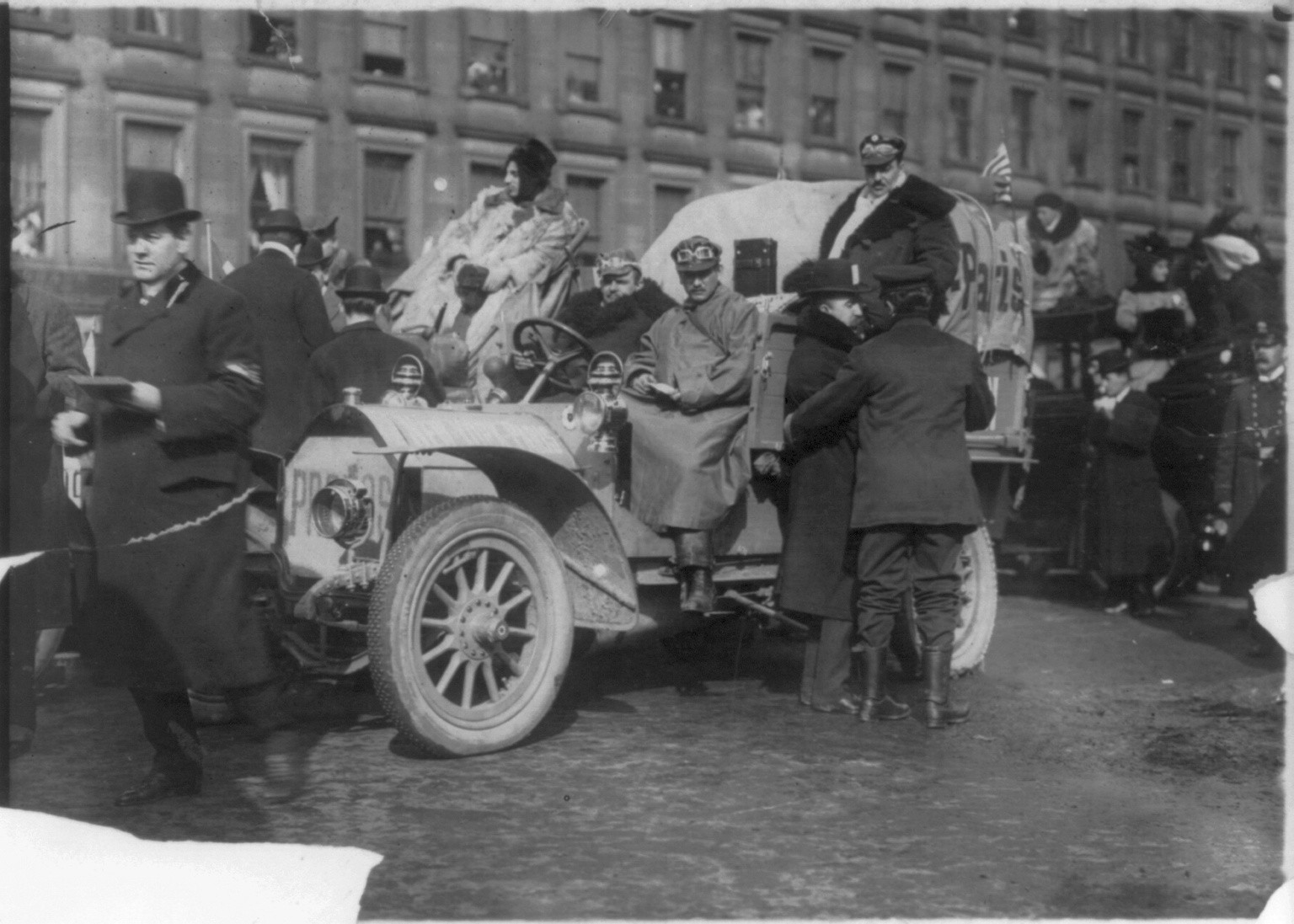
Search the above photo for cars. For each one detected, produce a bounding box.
[986,267,1207,605]
[274,133,1038,745]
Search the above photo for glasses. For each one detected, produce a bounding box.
[676,246,714,262]
[597,255,641,272]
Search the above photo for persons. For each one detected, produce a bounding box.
[5,132,992,807]
[1020,191,1288,662]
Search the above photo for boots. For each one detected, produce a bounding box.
[920,645,973,728]
[857,646,913,722]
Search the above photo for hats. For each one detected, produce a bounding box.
[1095,349,1134,372]
[799,259,877,295]
[1250,319,1288,347]
[251,209,307,246]
[671,234,723,272]
[296,238,330,268]
[1035,193,1063,209]
[859,132,907,165]
[110,167,203,225]
[508,137,557,181]
[595,247,643,278]
[334,265,389,305]
[314,216,340,243]
[873,264,934,299]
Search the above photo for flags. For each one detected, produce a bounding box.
[982,139,1014,204]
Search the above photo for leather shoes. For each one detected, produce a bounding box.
[679,568,720,611]
[811,690,862,714]
[114,766,203,807]
[263,729,314,806]
